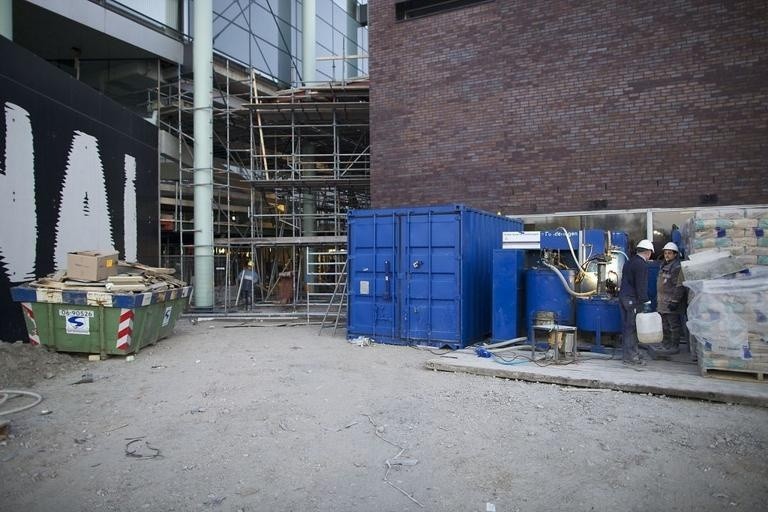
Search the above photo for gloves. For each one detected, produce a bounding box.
[642,304,653,313]
[666,302,678,312]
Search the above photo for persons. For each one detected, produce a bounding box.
[656,241,685,354]
[235,260,260,311]
[616,237,656,366]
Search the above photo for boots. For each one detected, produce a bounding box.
[661,327,682,355]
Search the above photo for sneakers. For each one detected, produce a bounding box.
[623,352,646,366]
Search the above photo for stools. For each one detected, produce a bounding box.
[532,324,577,364]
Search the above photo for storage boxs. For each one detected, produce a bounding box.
[67,250,120,282]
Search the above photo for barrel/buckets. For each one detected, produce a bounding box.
[634,302,664,344]
[528,268,577,344]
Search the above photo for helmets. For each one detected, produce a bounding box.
[247,260,255,267]
[636,239,655,252]
[662,241,680,253]
